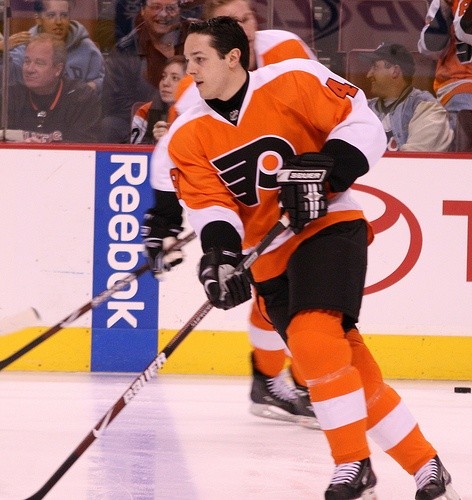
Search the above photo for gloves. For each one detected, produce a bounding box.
[274,153,330,225]
[198,249,253,311]
[140,201,186,273]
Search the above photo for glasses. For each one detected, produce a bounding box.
[142,3,179,13]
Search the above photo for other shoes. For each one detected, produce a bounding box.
[412,453,450,499]
[249,371,332,433]
[325,458,376,499]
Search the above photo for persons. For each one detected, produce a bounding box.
[0,0,471,157]
[135,0,319,421]
[169,16,451,500]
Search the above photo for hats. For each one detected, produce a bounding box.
[360,44,417,73]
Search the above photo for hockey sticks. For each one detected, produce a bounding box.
[0,212,291,500]
